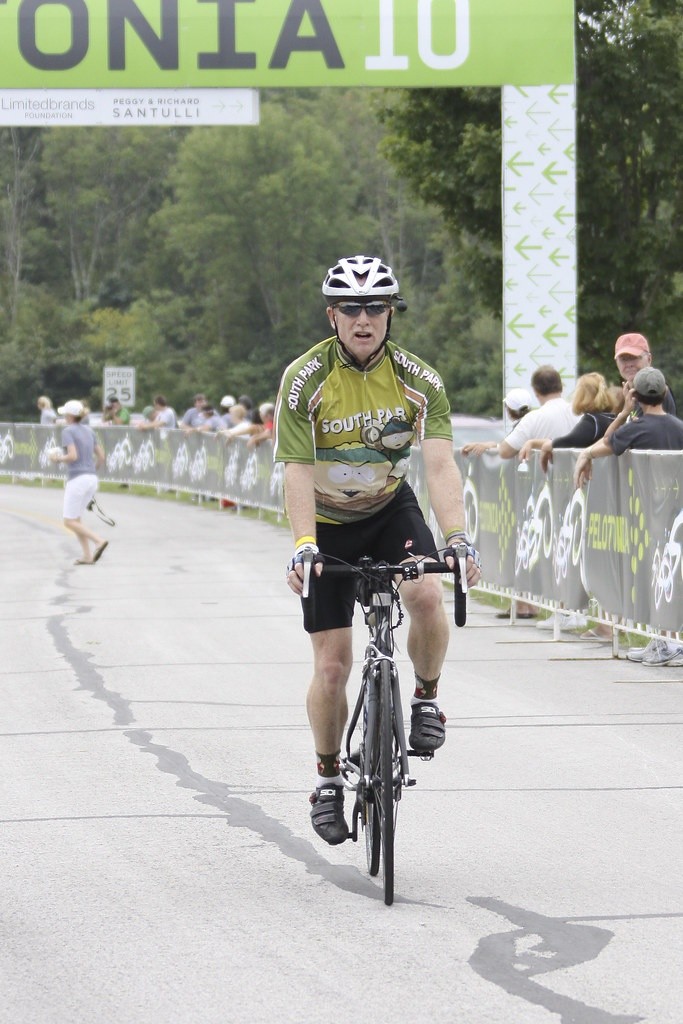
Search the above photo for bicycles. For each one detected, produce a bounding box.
[302,546,469,904]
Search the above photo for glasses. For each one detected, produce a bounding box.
[332,300,390,317]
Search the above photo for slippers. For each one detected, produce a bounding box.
[93,542,108,563]
[496,607,538,618]
[580,630,616,643]
[72,559,93,565]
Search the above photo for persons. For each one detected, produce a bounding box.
[37,393,275,449]
[49,400,108,565]
[272,255,482,844]
[462,333,683,668]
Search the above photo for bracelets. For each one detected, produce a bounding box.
[444,528,472,546]
[295,536,316,549]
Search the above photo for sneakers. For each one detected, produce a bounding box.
[641,644,683,666]
[541,610,587,630]
[627,640,654,662]
[408,702,446,751]
[309,781,349,846]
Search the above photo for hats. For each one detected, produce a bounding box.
[57,400,84,417]
[220,394,236,408]
[259,403,274,416]
[613,333,649,359]
[633,366,665,396]
[143,406,153,421]
[501,389,532,412]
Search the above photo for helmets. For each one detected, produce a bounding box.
[322,255,399,306]
